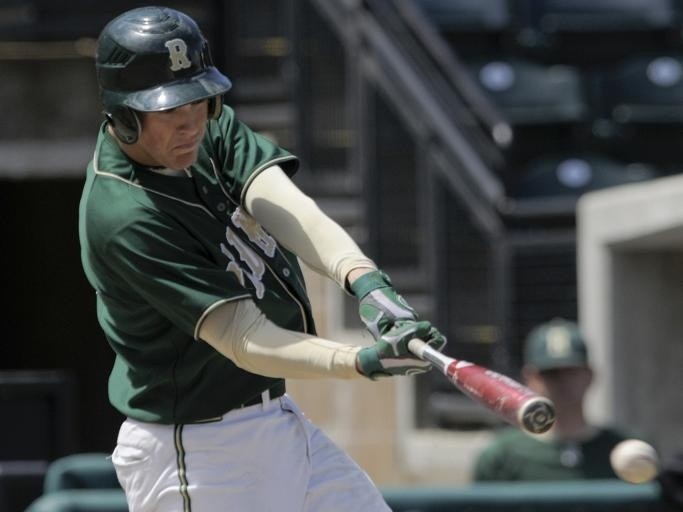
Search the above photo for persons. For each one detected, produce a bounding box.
[465,315,654,483]
[74,6,448,511]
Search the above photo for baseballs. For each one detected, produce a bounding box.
[610,440,660,483]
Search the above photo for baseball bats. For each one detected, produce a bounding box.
[407,338,557,436]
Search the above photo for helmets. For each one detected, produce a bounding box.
[92,3,235,148]
[516,314,593,375]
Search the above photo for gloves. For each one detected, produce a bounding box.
[353,319,450,380]
[346,268,423,343]
